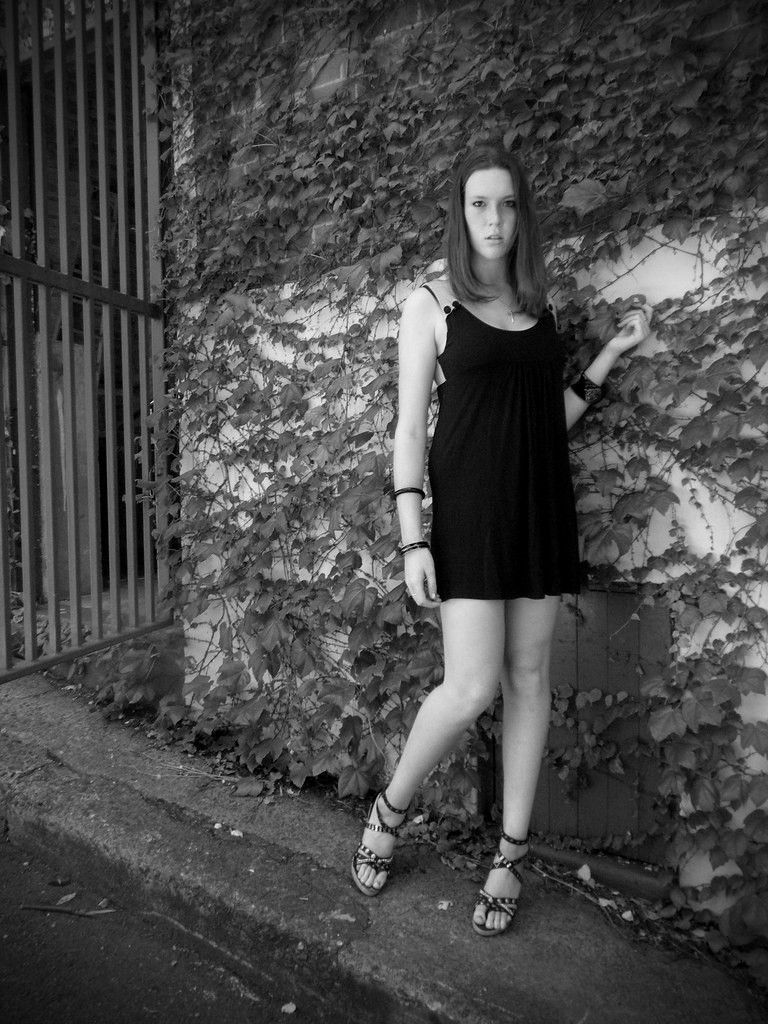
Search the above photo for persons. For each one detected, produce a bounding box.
[354,148,652,935]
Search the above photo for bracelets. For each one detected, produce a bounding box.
[570,372,602,403]
[399,541,432,556]
[392,486,426,500]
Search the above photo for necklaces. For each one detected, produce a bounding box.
[496,290,520,323]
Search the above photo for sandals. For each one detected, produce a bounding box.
[472,825,531,936]
[351,788,411,898]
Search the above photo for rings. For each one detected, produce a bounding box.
[643,318,648,321]
